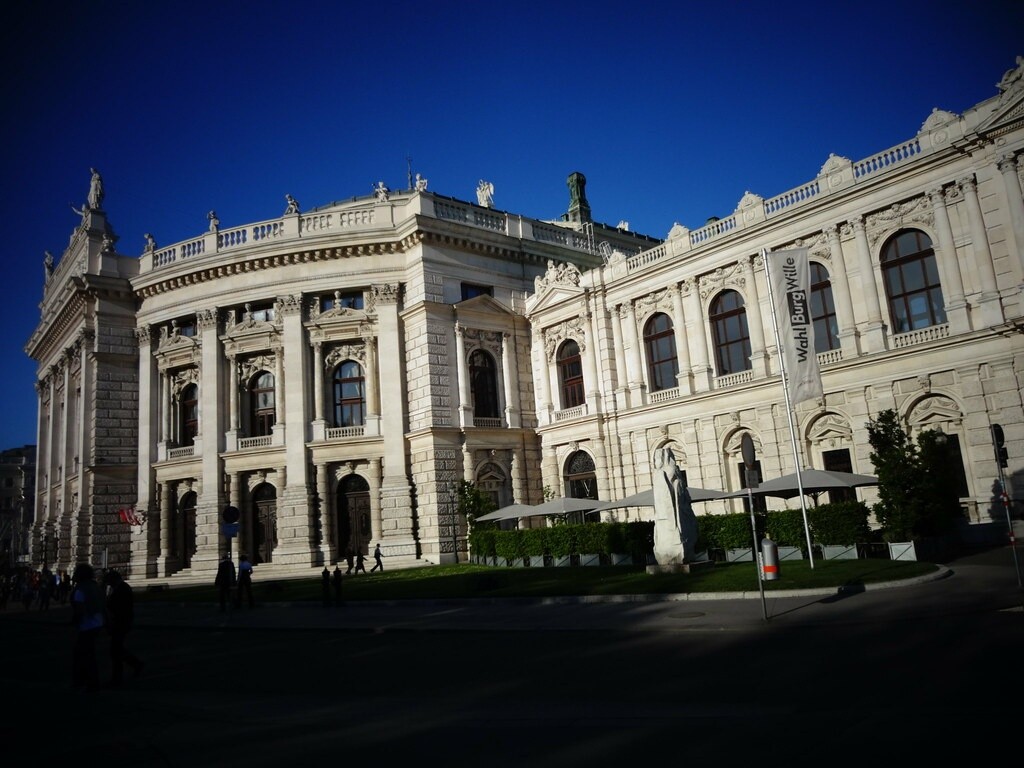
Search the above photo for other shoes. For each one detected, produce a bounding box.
[371,569,374,572]
[101,664,145,688]
[364,571,367,574]
[346,570,351,575]
[67,675,94,693]
[355,571,358,576]
[381,569,383,571]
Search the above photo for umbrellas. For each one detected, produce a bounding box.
[492,498,609,526]
[584,487,755,515]
[474,503,535,531]
[715,469,879,508]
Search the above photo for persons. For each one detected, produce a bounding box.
[355,546,367,575]
[334,291,343,303]
[42,250,54,284]
[171,320,179,332]
[284,194,299,214]
[345,548,354,575]
[235,555,255,608]
[0,563,137,693]
[100,234,116,253]
[71,167,104,222]
[143,232,154,252]
[375,181,387,197]
[244,303,254,317]
[214,553,236,611]
[414,174,428,192]
[532,261,580,298]
[206,210,220,231]
[370,543,385,573]
